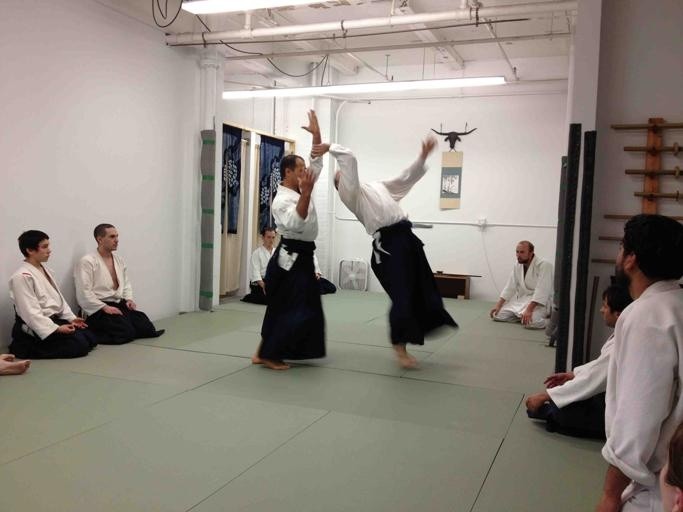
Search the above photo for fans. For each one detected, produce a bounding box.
[337,259,368,291]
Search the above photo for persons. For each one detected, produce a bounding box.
[658,421,682,512]
[0,353,31,375]
[311,136,460,369]
[73,223,165,345]
[526,284,633,441]
[595,213,682,512]
[312,249,336,295]
[490,241,555,328]
[7,230,97,358]
[252,109,322,369]
[240,227,277,305]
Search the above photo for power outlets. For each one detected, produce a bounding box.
[479,218,486,227]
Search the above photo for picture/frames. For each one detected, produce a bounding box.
[440,152,463,209]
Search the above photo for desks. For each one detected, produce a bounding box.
[434,273,482,299]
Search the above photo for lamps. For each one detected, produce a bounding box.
[221,51,508,100]
